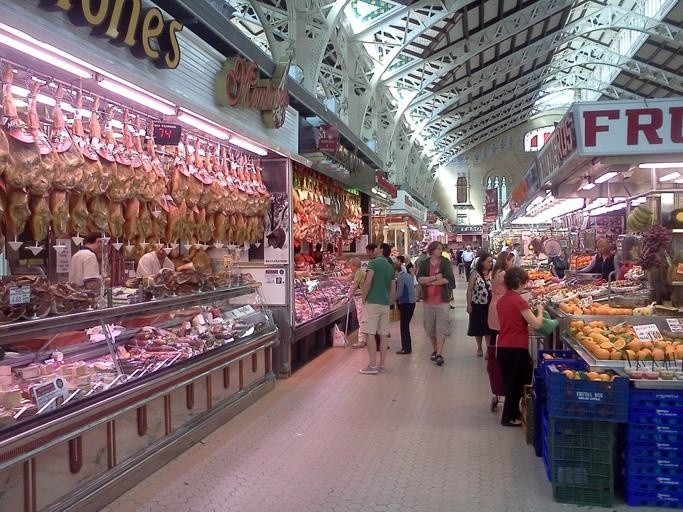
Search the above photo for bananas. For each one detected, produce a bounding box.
[627,204,652,231]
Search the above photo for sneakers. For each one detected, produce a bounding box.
[435,355,444,365]
[430,352,437,361]
[377,365,384,373]
[351,341,365,348]
[501,419,521,426]
[359,364,378,374]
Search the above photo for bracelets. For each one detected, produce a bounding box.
[435,276,437,280]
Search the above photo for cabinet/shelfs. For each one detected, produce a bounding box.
[292,266,357,370]
[0,284,277,509]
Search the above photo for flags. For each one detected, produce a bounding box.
[483,189,499,218]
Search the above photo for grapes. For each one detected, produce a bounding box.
[637,224,669,270]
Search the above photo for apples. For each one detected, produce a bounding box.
[633,307,651,316]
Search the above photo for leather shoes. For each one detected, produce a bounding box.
[396,348,409,354]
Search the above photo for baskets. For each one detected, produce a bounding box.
[531,351,682,509]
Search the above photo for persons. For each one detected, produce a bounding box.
[441,245,475,309]
[416,240,456,364]
[467,243,521,356]
[133,244,176,278]
[359,242,416,375]
[67,231,112,290]
[335,257,367,347]
[551,247,570,279]
[414,252,427,302]
[294,240,337,277]
[580,239,616,282]
[496,266,545,428]
